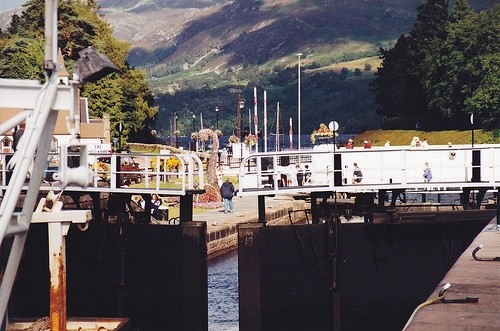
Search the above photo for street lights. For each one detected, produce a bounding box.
[238,95,246,164]
[297,52,302,168]
[214,104,219,130]
[191,112,197,151]
[175,112,179,148]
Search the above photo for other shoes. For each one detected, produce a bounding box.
[225,210,233,214]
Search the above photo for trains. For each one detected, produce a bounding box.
[237,143,500,194]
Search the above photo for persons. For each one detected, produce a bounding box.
[220,178,236,214]
[138,194,162,220]
[11,124,81,184]
[296,136,432,186]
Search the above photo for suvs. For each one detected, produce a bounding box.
[92,155,142,185]
